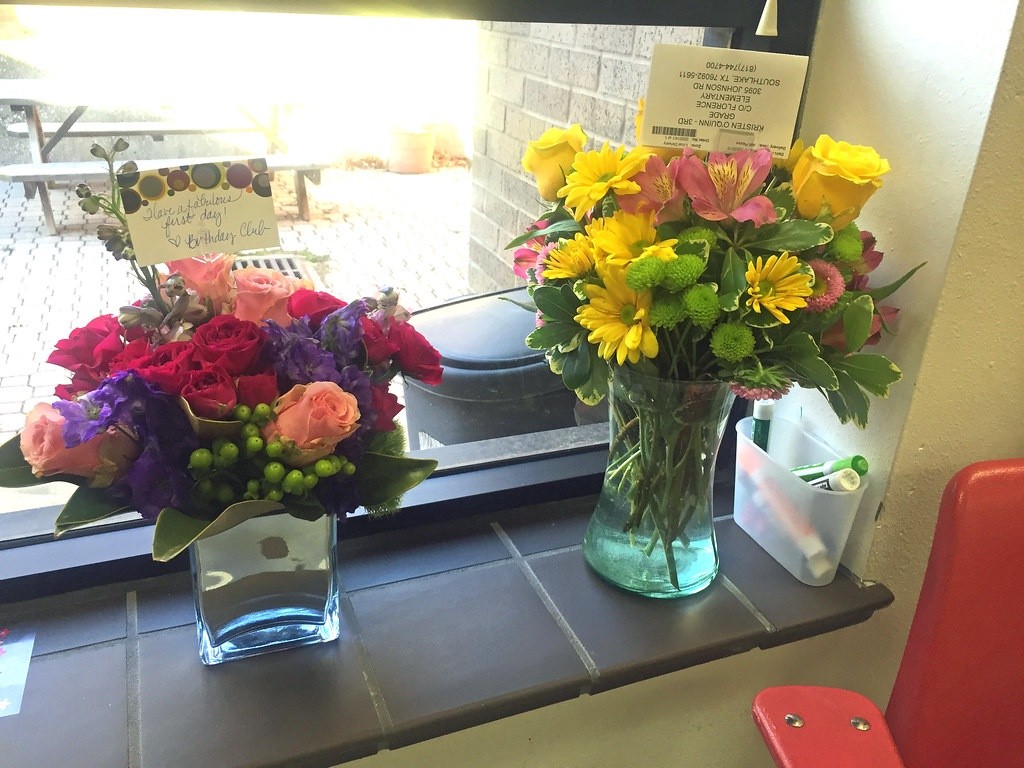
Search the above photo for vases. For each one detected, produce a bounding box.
[183,504,340,665]
[580,364,742,602]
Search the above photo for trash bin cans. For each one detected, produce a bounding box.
[395,282,593,452]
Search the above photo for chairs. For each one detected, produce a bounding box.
[754,458,1024,768]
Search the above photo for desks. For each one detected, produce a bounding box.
[0,78,353,235]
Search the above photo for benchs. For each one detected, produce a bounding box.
[1,148,328,185]
[6,121,286,137]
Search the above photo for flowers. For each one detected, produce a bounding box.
[0,136,444,562]
[500,98,933,590]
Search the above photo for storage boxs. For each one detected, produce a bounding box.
[733,415,871,588]
